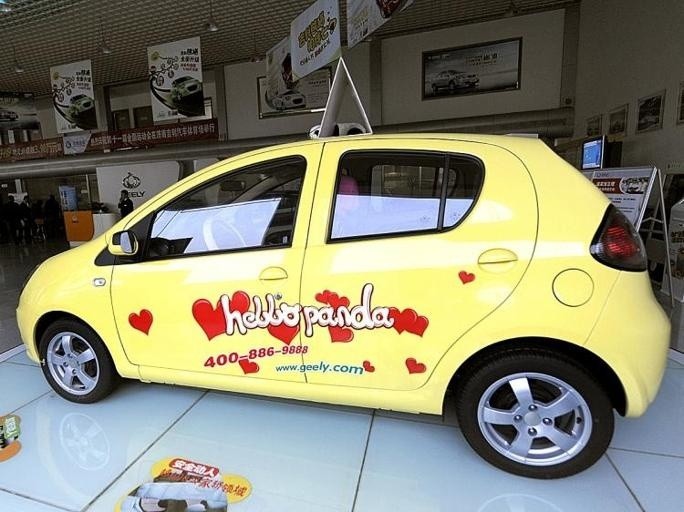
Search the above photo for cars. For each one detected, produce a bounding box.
[14,129,673,478]
[170,76,202,102]
[265,88,306,111]
[68,94,94,118]
[431,69,479,97]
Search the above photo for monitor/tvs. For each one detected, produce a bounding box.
[582,134,608,169]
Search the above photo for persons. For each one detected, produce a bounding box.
[117,190,134,218]
[3,190,63,246]
